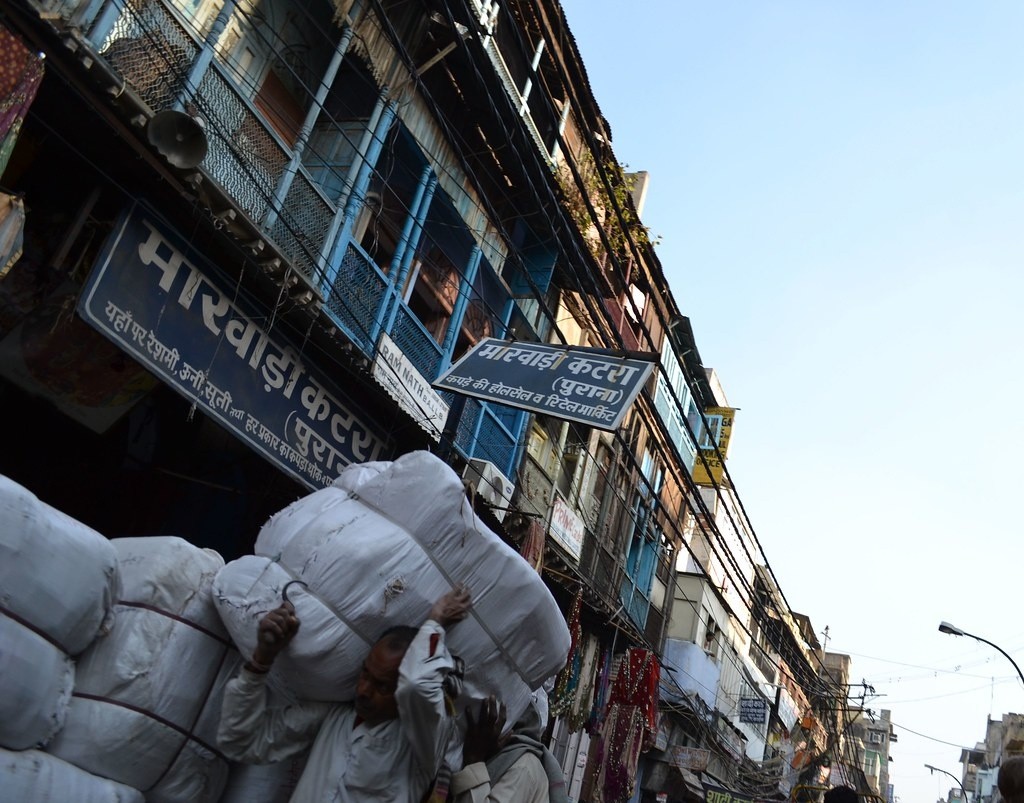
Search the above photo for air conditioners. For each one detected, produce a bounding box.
[459,458,516,523]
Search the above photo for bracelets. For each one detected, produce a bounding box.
[244,662,269,674]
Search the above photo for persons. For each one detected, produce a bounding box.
[452,686,567,803]
[996,756,1024,803]
[215,585,473,803]
[823,786,857,803]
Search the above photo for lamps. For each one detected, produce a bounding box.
[143,111,214,171]
